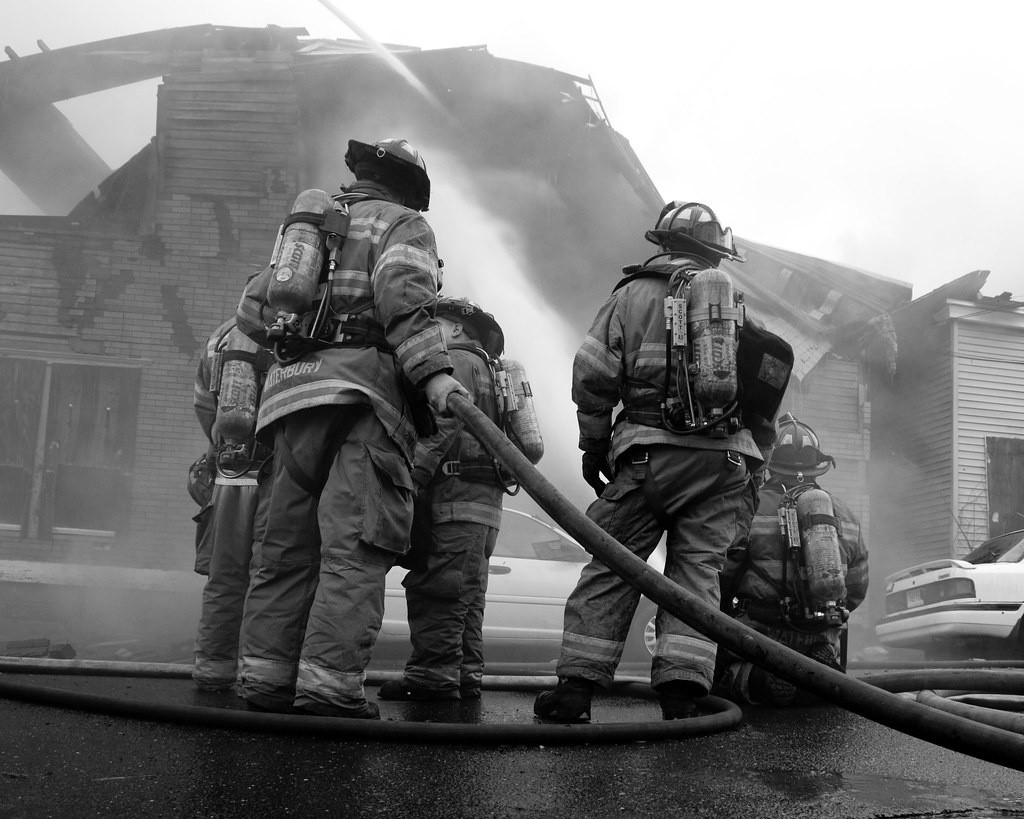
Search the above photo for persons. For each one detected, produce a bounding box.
[187,270,270,695]
[530,201,791,722]
[710,414,869,705]
[232,138,468,717]
[377,298,502,701]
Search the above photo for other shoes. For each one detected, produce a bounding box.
[660,691,702,721]
[377,677,481,699]
[307,702,381,720]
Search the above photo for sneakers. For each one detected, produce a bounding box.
[533,680,591,722]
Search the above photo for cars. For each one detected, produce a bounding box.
[874,524,1024,659]
[375,501,666,659]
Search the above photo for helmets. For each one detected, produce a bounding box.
[437,296,505,359]
[645,201,747,263]
[345,138,430,213]
[767,411,835,478]
